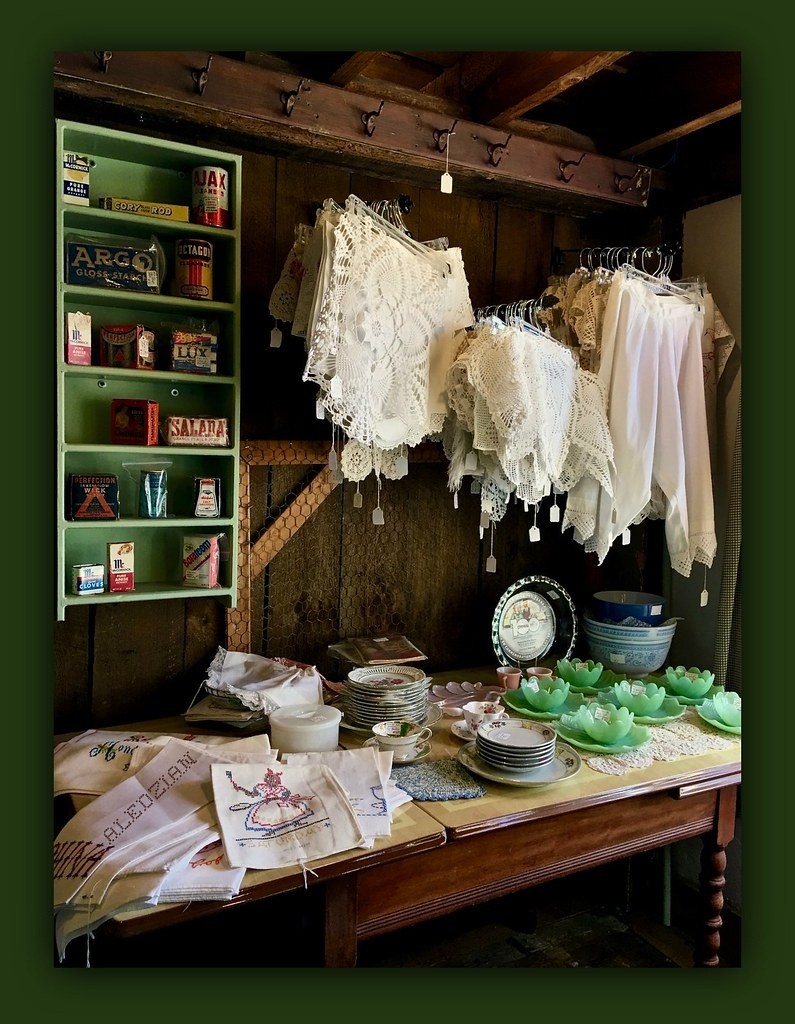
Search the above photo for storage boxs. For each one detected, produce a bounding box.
[99,197,191,222]
[182,532,219,587]
[105,541,135,593]
[68,472,120,521]
[64,309,216,376]
[65,240,161,294]
[109,397,159,446]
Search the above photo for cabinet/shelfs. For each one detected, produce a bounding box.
[53,116,244,623]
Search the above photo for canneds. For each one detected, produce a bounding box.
[194,167,228,228]
[174,239,213,300]
[139,470,167,518]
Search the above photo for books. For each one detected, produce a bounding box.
[186,698,262,728]
[328,634,428,665]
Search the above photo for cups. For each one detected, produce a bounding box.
[462,701,509,737]
[497,667,522,690]
[373,720,432,759]
[527,667,558,682]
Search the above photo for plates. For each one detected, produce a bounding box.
[451,670,741,787]
[362,736,432,764]
[331,666,443,732]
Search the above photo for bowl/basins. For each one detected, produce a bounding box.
[269,704,341,762]
[578,704,634,744]
[583,610,677,641]
[584,625,673,680]
[557,658,603,686]
[521,676,570,711]
[593,590,667,628]
[492,576,577,678]
[713,692,741,727]
[615,681,665,715]
[666,666,715,698]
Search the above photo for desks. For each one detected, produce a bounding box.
[54,665,742,970]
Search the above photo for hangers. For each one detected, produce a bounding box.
[362,192,705,332]
